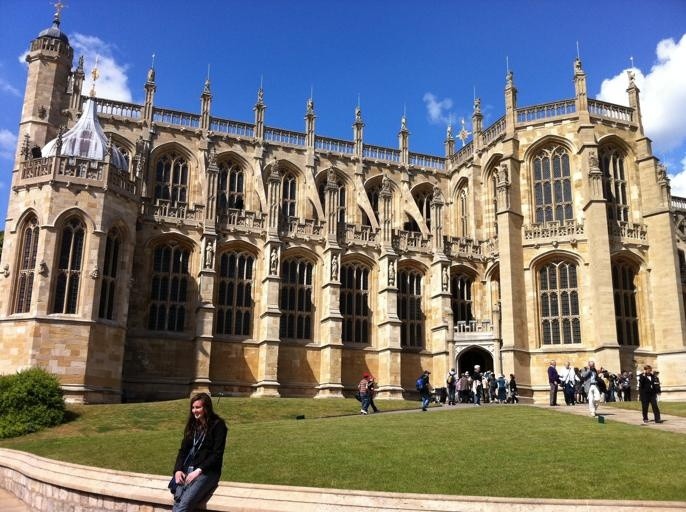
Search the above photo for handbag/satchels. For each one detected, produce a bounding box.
[168,466,189,493]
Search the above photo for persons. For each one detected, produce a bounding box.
[367,376,381,412]
[421,370,434,410]
[446,365,519,405]
[170,392,228,511]
[357,372,371,414]
[547,359,635,407]
[639,364,663,423]
[652,369,662,403]
[581,360,602,417]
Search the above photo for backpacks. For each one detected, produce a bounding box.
[415,378,425,391]
[447,372,457,384]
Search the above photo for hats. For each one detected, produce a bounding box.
[363,373,376,379]
